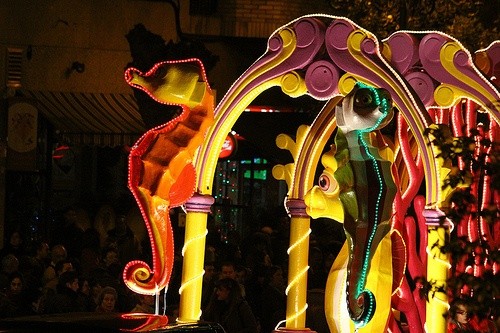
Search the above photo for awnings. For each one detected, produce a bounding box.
[20,87,144,151]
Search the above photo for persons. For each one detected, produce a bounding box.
[105,214,131,246]
[0,225,338,315]
[204,277,257,333]
[249,266,286,332]
[51,206,81,239]
